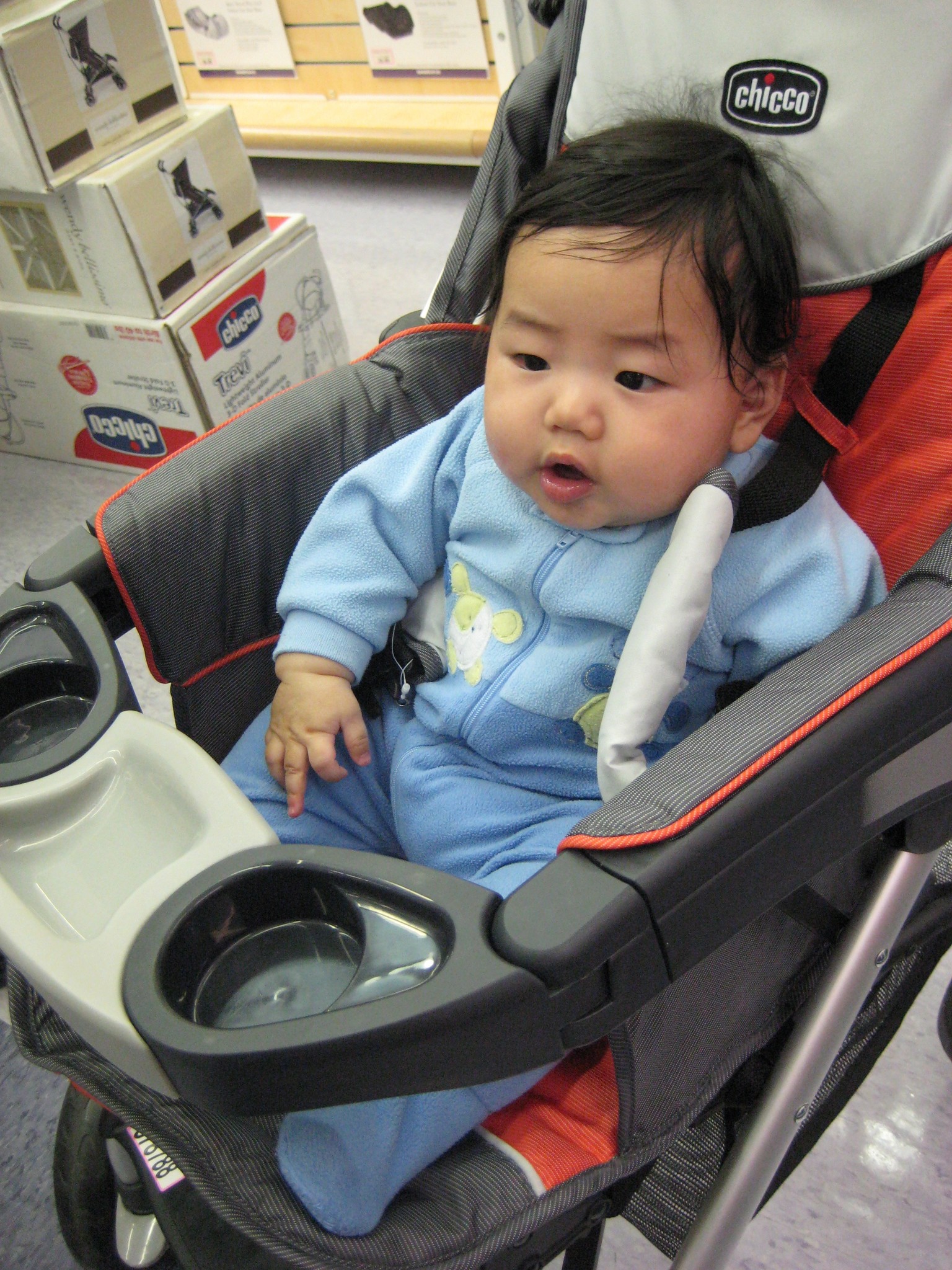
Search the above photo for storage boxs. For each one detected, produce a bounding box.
[0,100,272,320]
[0,0,193,196]
[0,210,354,475]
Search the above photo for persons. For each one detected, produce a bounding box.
[222,78,887,1239]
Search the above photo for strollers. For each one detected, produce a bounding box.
[0,1,952,1270]
